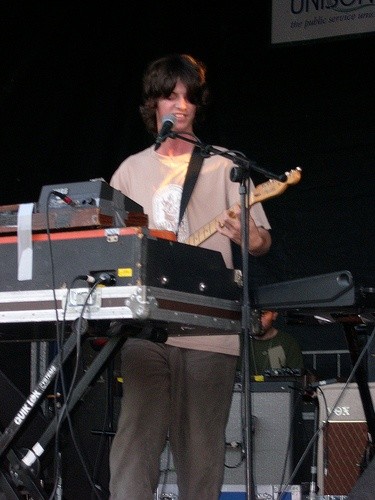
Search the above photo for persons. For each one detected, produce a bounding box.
[231,291,308,385]
[106,53,271,500]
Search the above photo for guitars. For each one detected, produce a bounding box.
[84,166,303,353]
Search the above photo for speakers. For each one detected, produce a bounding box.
[156,379,295,488]
[316,387,375,497]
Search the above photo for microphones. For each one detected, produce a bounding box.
[241,416,256,456]
[154,114,177,152]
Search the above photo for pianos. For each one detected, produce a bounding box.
[1,225,243,500]
